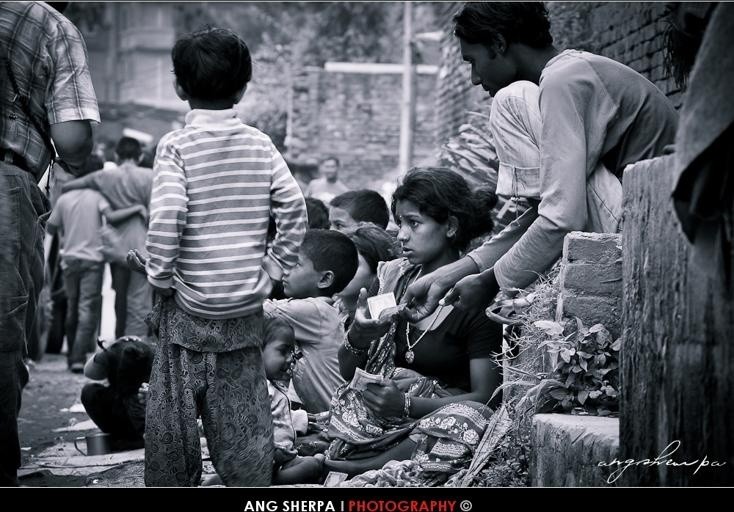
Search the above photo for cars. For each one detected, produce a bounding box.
[73,432,110,456]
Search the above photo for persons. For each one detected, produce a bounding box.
[0,0,734,485]
